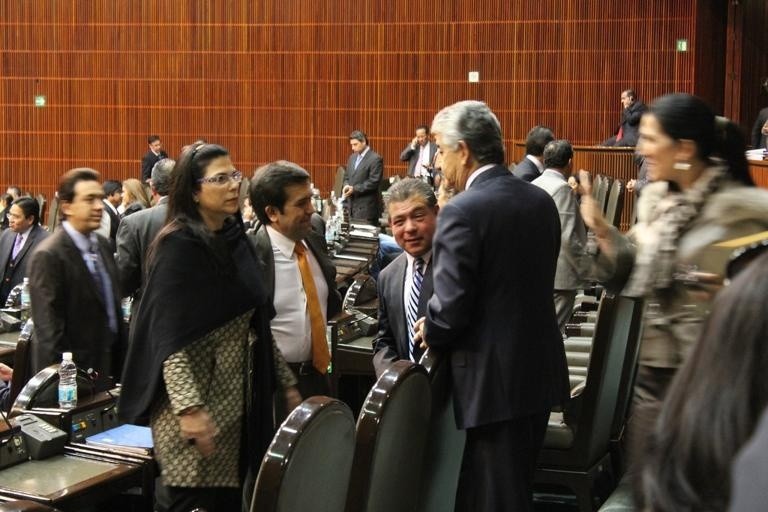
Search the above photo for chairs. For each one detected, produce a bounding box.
[0,171,645,505]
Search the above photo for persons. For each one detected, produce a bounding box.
[0,89,768,512]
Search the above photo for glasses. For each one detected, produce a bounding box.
[195,170,244,185]
[6,213,18,220]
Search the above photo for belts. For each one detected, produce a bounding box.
[287,361,314,377]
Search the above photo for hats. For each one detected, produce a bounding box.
[407,258,426,364]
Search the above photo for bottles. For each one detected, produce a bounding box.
[21,277,31,311]
[57,352,79,409]
[121,295,132,322]
[324,190,344,242]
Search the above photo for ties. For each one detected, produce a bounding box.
[11,232,23,259]
[355,154,362,165]
[413,145,424,177]
[87,245,105,297]
[294,241,332,376]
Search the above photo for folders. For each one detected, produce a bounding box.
[85,423,156,456]
